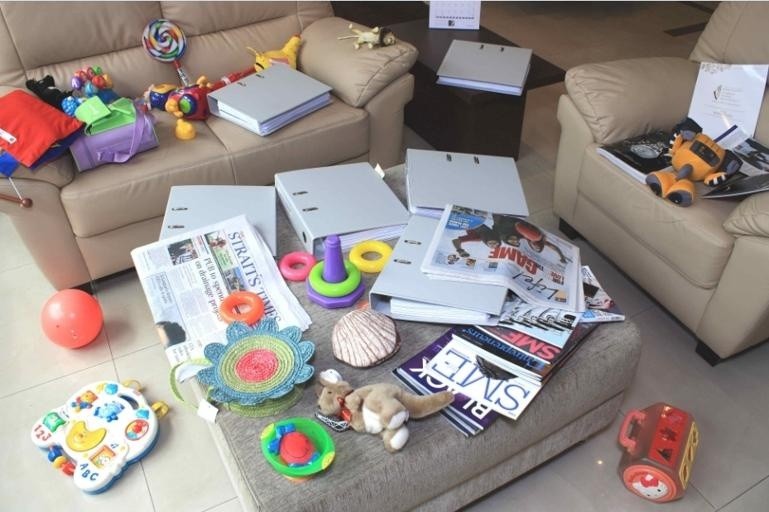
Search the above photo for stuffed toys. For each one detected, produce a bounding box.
[316,371,454,452]
[338,22,399,51]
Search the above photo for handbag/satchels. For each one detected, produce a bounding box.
[70,96,158,173]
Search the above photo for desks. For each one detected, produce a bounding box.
[383,18,565,162]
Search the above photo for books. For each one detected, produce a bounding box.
[392,265,625,437]
[596,130,674,184]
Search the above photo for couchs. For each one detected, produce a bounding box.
[1,1,420,296]
[552,2,768,368]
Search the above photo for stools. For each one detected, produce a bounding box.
[189,163,643,512]
